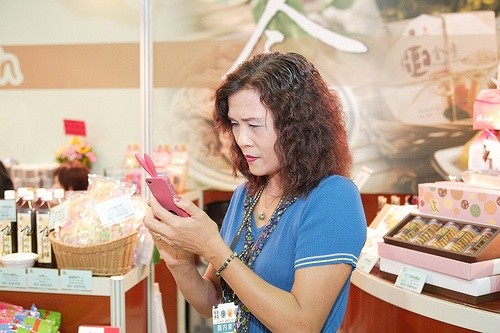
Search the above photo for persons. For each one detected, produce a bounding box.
[142,51,367,333]
[51,167,88,192]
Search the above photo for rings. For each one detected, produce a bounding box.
[156,234,161,241]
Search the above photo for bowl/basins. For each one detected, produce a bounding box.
[0,252,40,268]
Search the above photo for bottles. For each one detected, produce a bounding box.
[376,194,410,215]
[0,187,65,268]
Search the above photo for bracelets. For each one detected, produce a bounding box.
[216,253,238,278]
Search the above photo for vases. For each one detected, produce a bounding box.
[58,165,89,190]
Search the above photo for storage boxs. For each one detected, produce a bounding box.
[372,181,500,309]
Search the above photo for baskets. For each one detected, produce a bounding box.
[48,226,139,278]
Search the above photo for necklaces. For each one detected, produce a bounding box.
[227,184,301,333]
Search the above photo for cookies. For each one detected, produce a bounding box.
[394,216,494,255]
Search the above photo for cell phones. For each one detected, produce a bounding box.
[145,175,191,218]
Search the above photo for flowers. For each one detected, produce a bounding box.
[54,140,98,168]
